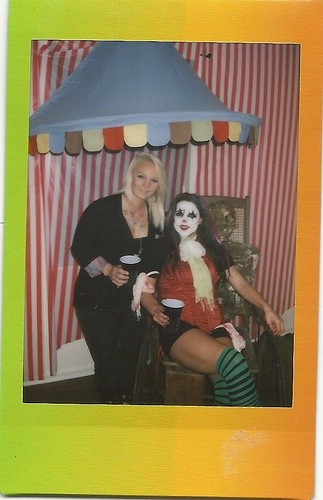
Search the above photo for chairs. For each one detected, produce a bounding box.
[133,194,284,407]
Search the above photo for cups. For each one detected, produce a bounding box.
[161,299,184,333]
[120,255,141,286]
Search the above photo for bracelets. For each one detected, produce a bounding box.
[103,263,112,275]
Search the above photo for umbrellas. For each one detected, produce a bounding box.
[29,41,262,154]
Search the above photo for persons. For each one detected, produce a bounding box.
[136,192,284,406]
[69,153,168,403]
